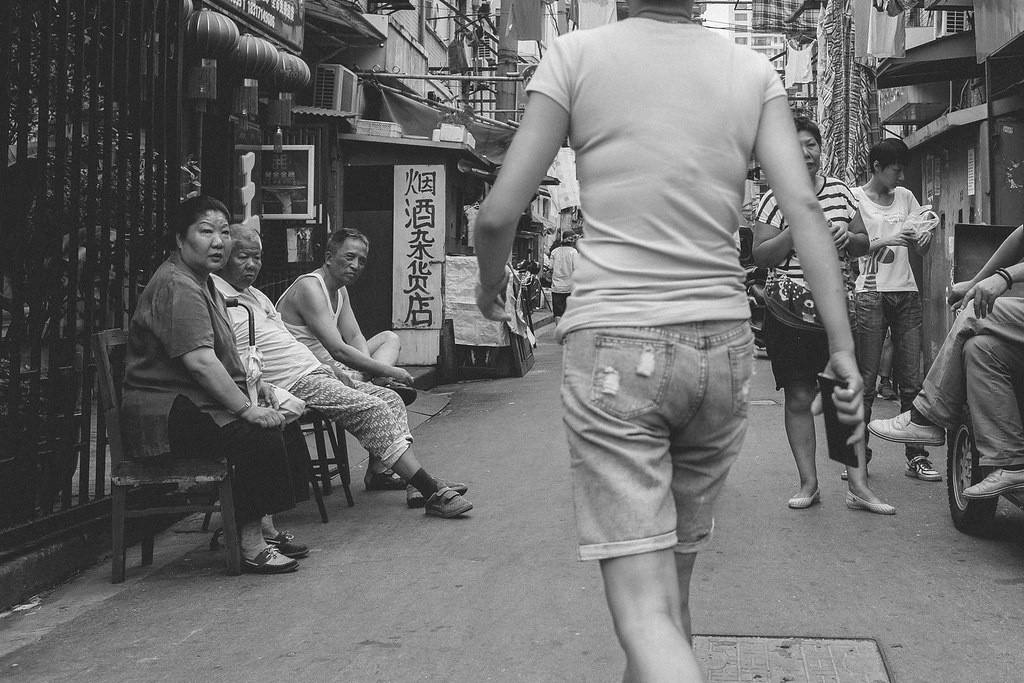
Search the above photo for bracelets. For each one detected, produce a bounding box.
[996,268,1013,290]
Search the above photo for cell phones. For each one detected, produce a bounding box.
[817,373,859,469]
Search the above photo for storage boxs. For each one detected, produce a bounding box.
[467,133,476,150]
[356,120,402,138]
[432,129,441,141]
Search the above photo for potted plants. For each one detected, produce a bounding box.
[435,105,476,144]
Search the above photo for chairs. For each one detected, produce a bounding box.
[89,327,244,584]
[200,408,354,531]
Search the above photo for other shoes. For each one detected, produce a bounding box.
[366,469,406,490]
[388,382,417,407]
[788,488,820,508]
[265,530,309,556]
[242,543,300,574]
[846,490,895,515]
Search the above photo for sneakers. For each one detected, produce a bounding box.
[905,456,942,481]
[841,470,848,479]
[407,477,468,508]
[425,486,473,518]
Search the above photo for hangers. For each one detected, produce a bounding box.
[785,32,818,44]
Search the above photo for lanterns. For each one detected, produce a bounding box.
[259,51,311,126]
[184,8,240,112]
[221,33,280,119]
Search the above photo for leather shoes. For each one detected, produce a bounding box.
[866,410,945,446]
[962,467,1024,500]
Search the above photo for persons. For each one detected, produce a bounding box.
[120,198,310,574]
[752,117,895,514]
[209,224,473,519]
[841,138,942,483]
[275,227,468,508]
[548,230,579,326]
[475,0,865,683]
[868,224,1024,498]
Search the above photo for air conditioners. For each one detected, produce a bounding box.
[932,10,967,37]
[312,63,358,113]
[471,56,489,84]
[478,29,497,59]
